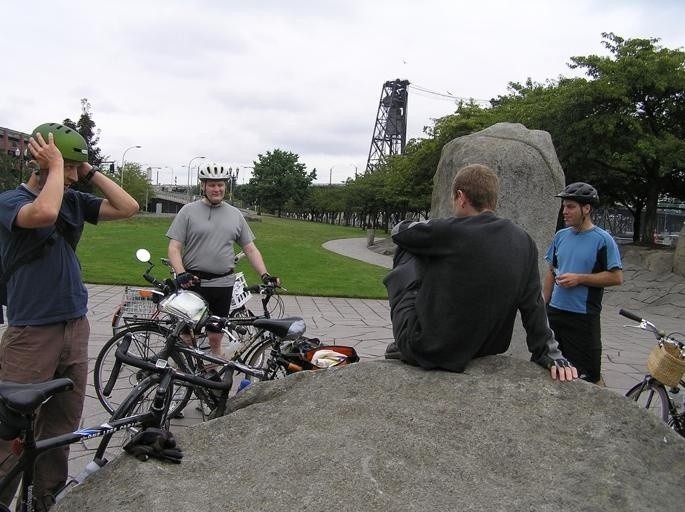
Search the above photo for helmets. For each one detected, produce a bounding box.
[554,182,600,207]
[199,164,230,180]
[23,123,89,162]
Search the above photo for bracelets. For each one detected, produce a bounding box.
[83,166,100,184]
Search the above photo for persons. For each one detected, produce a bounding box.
[165,164,280,419]
[543,180,624,388]
[1,121,141,511]
[382,163,578,382]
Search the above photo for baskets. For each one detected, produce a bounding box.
[230,271,252,310]
[121,289,158,320]
[648,340,685,387]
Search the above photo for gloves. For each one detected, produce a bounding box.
[123,427,182,464]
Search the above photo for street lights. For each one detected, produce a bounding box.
[165,166,174,177]
[228,166,240,205]
[144,167,163,211]
[120,146,141,188]
[15,148,28,183]
[187,157,206,203]
[244,166,255,169]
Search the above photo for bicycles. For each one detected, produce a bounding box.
[618,307,685,437]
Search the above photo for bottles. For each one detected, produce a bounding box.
[668,385,685,415]
[236,379,251,393]
[54,457,108,502]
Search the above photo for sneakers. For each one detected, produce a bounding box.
[386,342,402,358]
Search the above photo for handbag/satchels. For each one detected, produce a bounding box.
[304,346,359,369]
